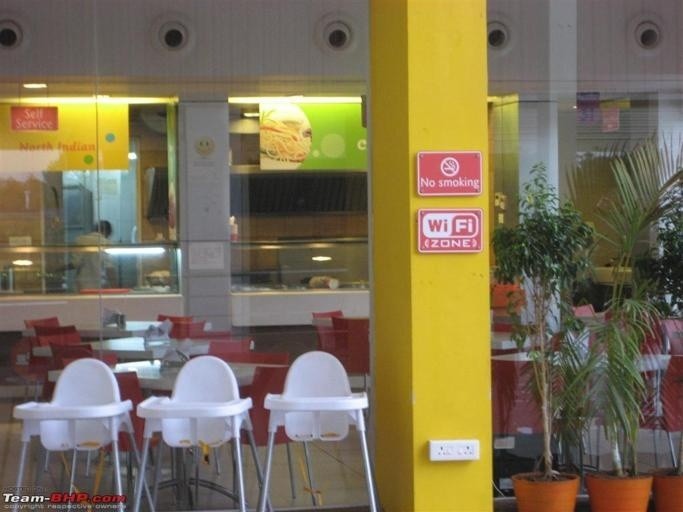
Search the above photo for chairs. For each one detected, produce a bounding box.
[126,353,273,512]
[493,278,681,468]
[7,356,156,511]
[113,370,159,495]
[226,364,294,505]
[257,349,378,512]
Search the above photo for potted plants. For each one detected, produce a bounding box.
[567,124,654,512]
[629,177,683,512]
[491,157,580,511]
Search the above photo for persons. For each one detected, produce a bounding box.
[54,220,119,294]
